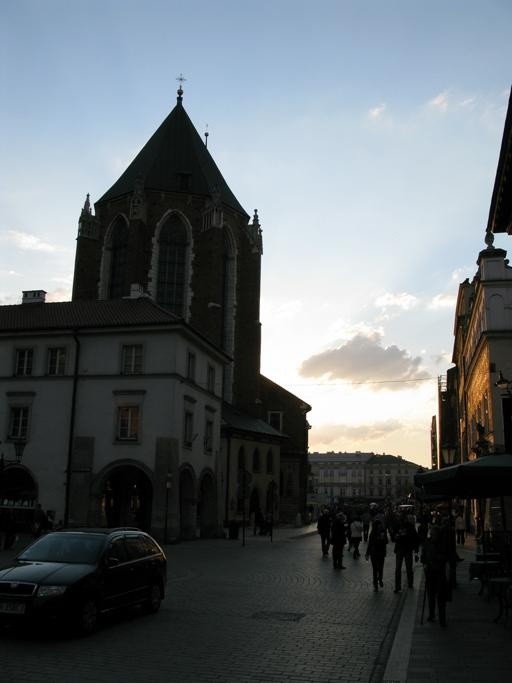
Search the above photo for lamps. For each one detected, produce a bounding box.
[495,369,510,389]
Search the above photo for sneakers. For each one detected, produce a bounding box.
[321,544,361,569]
[373,577,413,592]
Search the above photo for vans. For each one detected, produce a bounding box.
[338,496,386,523]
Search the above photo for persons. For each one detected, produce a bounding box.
[254,508,264,534]
[0,509,63,551]
[309,499,468,627]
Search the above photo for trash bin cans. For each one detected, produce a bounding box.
[230,520,238,539]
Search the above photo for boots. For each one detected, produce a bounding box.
[427,607,448,628]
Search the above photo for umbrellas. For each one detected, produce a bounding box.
[413,453,512,529]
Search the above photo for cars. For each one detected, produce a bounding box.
[0,526,166,639]
